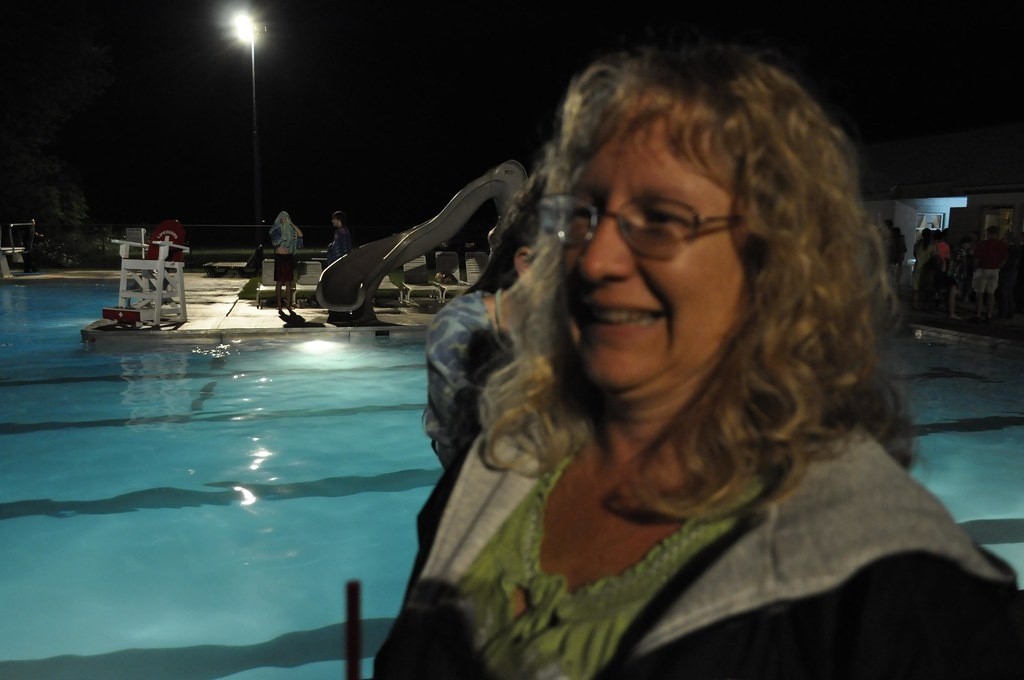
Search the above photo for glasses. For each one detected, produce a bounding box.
[533,193,745,257]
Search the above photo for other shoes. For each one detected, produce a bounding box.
[913,305,921,311]
[977,317,985,321]
[999,312,1013,318]
[949,312,962,320]
[986,316,993,322]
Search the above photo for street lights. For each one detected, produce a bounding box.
[227,12,268,230]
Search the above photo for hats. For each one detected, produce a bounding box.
[960,236,973,243]
[985,226,999,232]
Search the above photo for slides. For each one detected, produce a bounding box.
[315,160,530,312]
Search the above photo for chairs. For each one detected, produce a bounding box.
[401,254,442,308]
[465,251,489,285]
[433,251,470,303]
[111,220,190,326]
[377,276,400,305]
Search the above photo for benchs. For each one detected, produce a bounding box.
[257,259,323,310]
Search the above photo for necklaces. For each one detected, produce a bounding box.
[494,284,515,347]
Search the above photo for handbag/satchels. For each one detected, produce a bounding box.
[925,255,941,273]
[435,271,459,286]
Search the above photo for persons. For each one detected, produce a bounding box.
[373,45,1023,680]
[23,219,44,274]
[881,219,1023,323]
[327,211,352,266]
[423,161,544,478]
[269,211,303,310]
